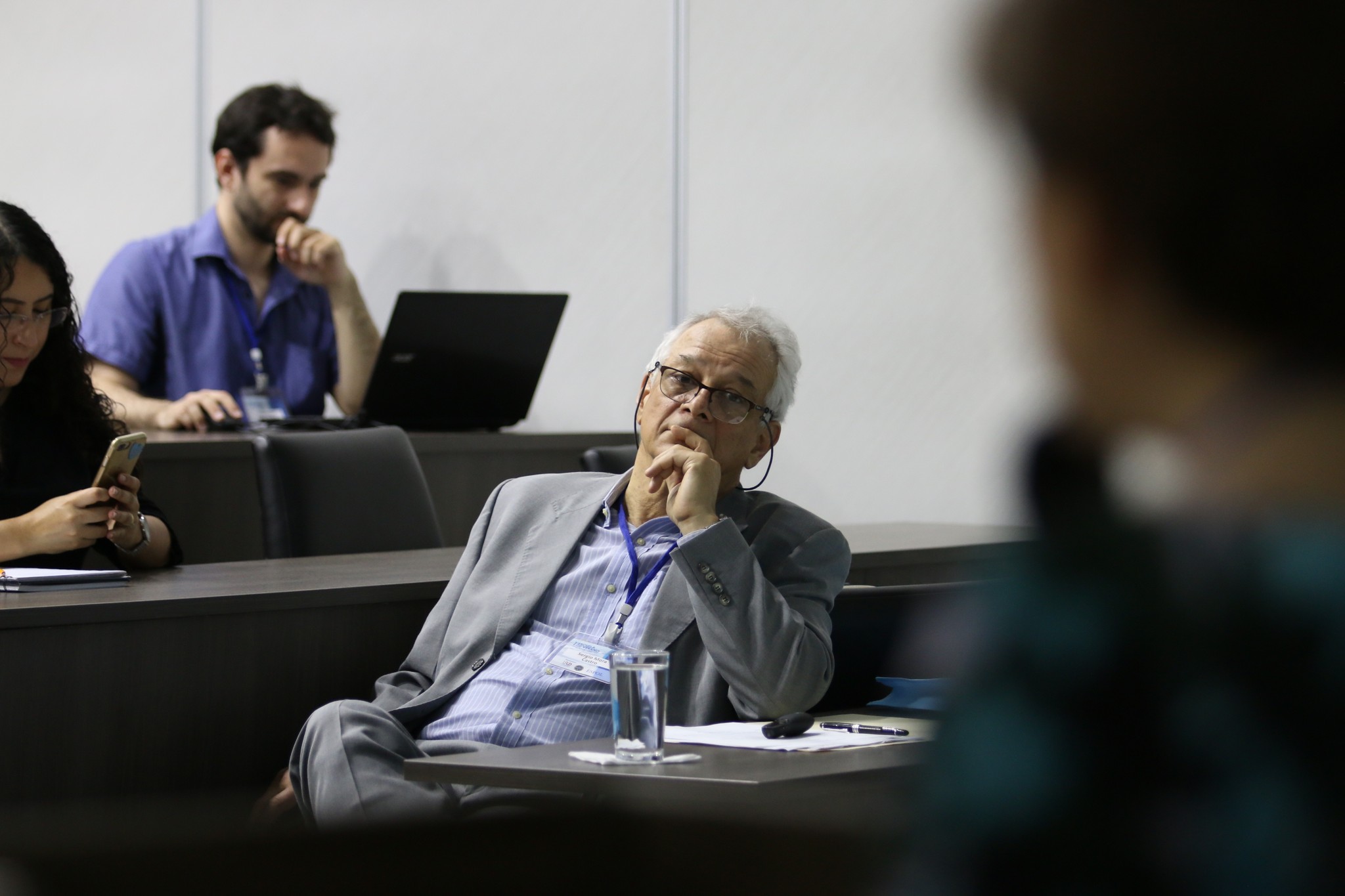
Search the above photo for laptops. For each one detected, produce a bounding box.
[260,290,569,431]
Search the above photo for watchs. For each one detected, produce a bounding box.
[115,511,152,556]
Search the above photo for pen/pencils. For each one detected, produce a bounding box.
[820,722,909,737]
[0,569,5,577]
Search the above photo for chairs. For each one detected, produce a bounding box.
[583,442,636,472]
[687,583,1003,726]
[254,426,441,557]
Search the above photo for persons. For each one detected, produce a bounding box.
[251,305,853,842]
[0,203,182,574]
[74,83,381,430]
[872,2,1345,896]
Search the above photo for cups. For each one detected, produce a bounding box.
[608,648,670,759]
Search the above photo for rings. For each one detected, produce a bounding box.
[124,513,136,528]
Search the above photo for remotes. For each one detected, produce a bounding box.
[762,712,815,739]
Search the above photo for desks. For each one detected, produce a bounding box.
[1,430,928,816]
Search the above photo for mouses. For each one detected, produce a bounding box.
[192,407,243,431]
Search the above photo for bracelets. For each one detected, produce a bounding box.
[701,513,728,530]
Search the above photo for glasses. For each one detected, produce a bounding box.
[0,307,69,332]
[655,361,772,424]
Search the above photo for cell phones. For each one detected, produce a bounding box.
[91,432,147,490]
